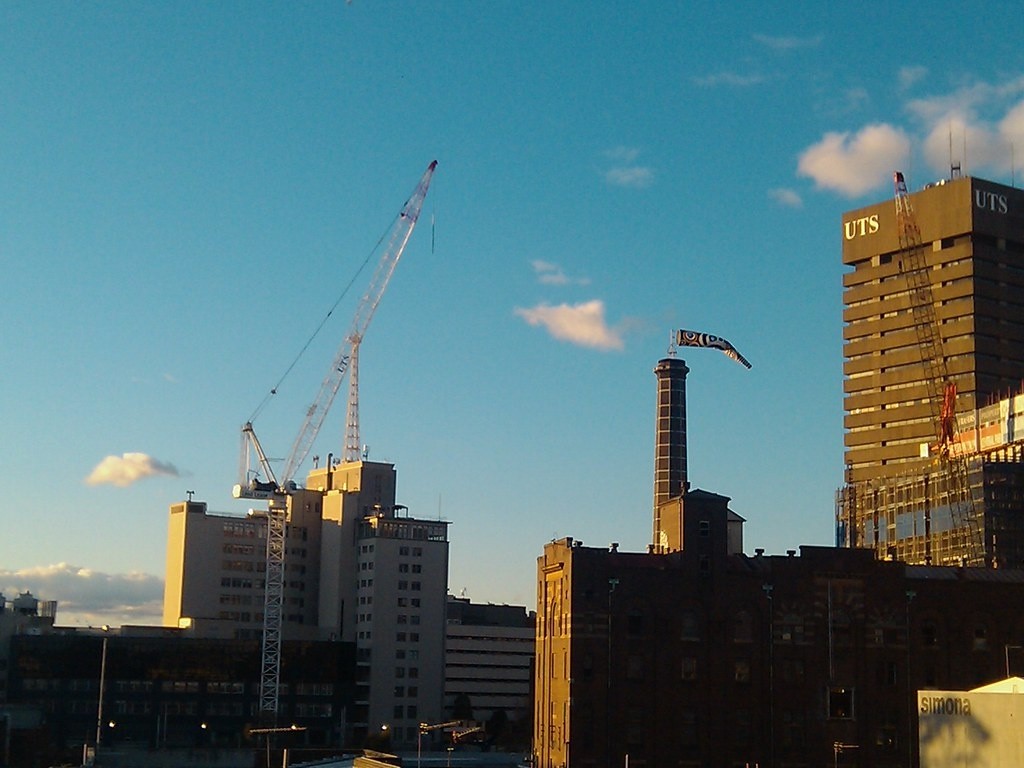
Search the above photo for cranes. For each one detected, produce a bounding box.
[891,169,985,563]
[231,158,441,498]
